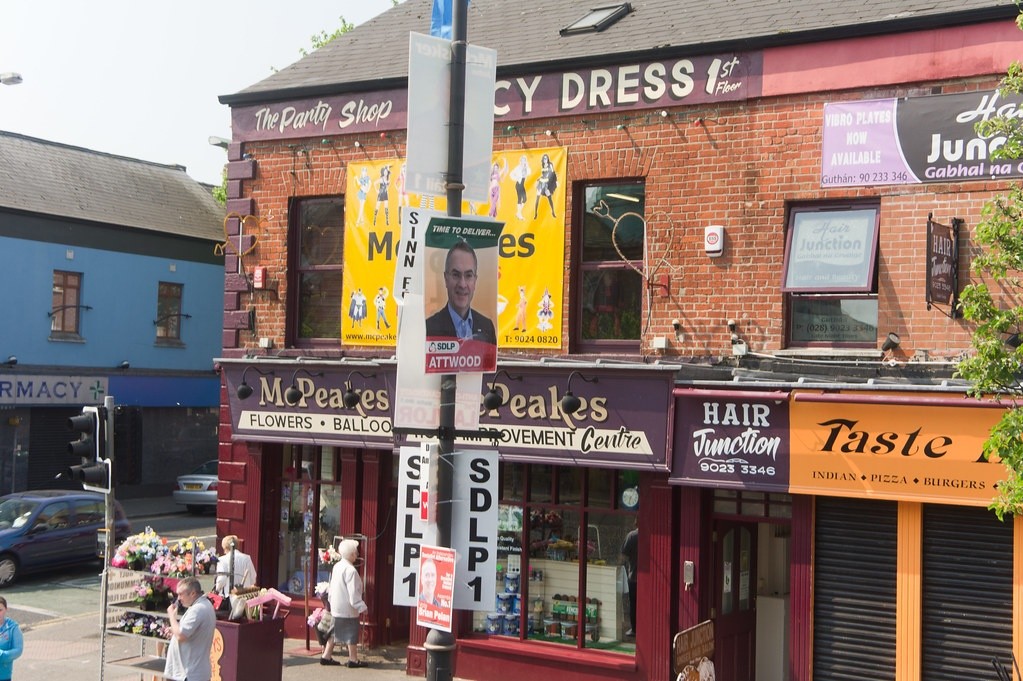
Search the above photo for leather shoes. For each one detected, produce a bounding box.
[348,661,368,667]
[320,657,340,665]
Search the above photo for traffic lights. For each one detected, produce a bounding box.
[68,457,111,495]
[66,405,101,464]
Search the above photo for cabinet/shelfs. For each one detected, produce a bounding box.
[98,532,237,680]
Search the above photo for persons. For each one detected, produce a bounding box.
[513,289,528,332]
[487,156,508,218]
[620,516,639,636]
[163,577,216,681]
[510,156,530,221]
[356,167,369,224]
[319,540,370,668]
[348,288,367,328]
[426,242,497,345]
[394,162,409,224]
[0,596,24,681]
[373,165,390,225]
[533,155,559,219]
[215,536,257,598]
[373,286,391,329]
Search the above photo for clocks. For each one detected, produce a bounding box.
[620,484,640,511]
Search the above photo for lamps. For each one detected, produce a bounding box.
[343,369,377,408]
[286,368,324,404]
[237,366,274,401]
[4,355,18,366]
[560,371,599,416]
[482,369,523,410]
[116,361,129,369]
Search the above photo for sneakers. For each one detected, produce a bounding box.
[625,627,635,636]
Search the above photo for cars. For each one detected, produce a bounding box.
[173,460,219,511]
[0,491,131,588]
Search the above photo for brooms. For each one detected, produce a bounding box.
[227,584,294,624]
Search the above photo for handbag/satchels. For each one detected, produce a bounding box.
[314,610,335,646]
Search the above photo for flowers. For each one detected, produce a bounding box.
[111,526,221,640]
